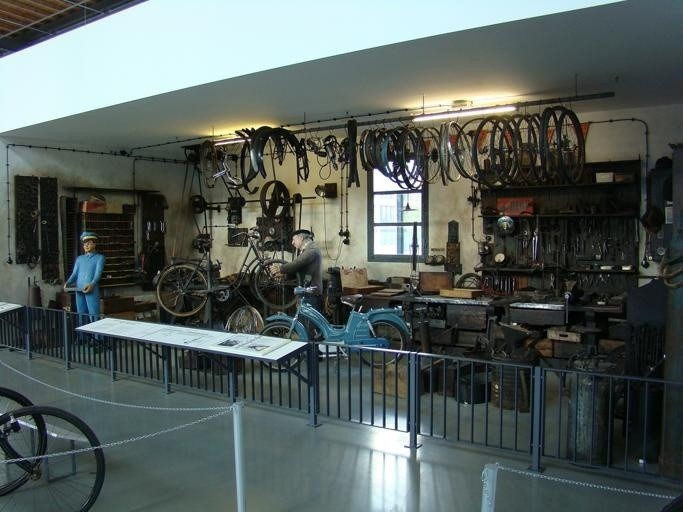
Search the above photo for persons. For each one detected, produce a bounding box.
[63,232,106,355]
[269,229,323,362]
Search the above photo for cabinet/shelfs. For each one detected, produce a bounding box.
[355,293,626,396]
[480,158,639,301]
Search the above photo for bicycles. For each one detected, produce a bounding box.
[0,387,106,512]
[154,226,302,319]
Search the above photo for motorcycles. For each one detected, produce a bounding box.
[257,274,412,373]
